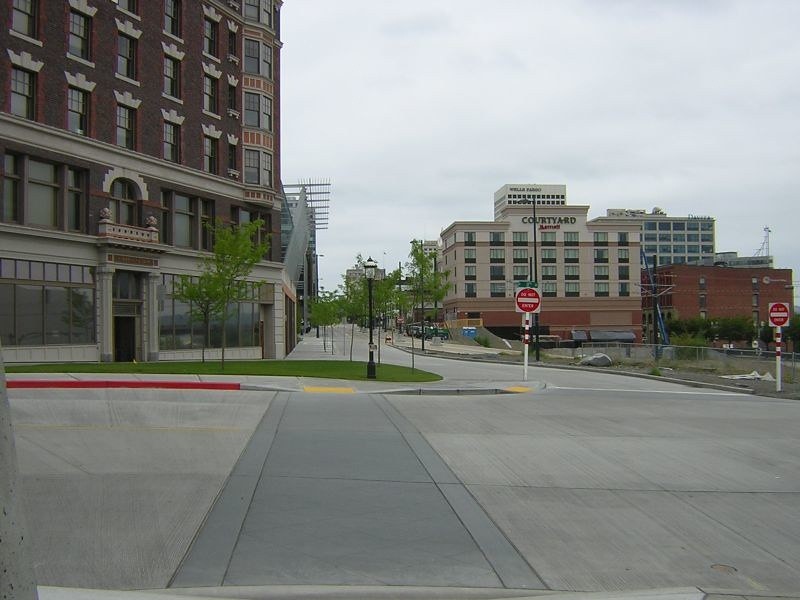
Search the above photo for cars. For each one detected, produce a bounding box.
[406,324,449,341]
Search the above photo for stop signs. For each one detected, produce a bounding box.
[769,303,790,327]
[515,288,541,313]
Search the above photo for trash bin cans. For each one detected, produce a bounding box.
[463,326,477,336]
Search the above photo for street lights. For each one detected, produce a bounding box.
[364,256,378,378]
[410,240,425,350]
[518,197,540,362]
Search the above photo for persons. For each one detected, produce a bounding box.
[306,320,311,333]
